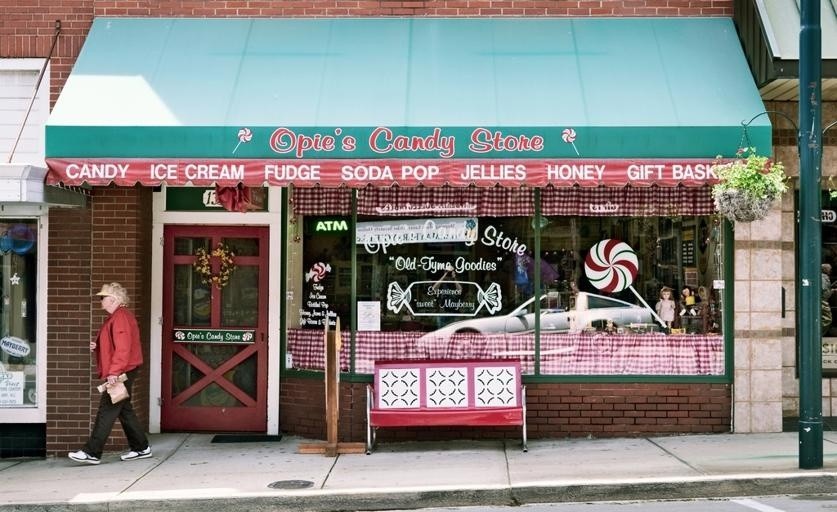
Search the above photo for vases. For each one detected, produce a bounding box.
[711,185,775,223]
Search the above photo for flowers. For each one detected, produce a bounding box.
[709,143,794,211]
[192,238,239,291]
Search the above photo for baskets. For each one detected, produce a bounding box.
[720,187,775,222]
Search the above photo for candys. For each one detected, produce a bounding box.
[387,280,502,316]
[585,237,670,330]
[561,128,579,155]
[231,128,252,154]
[306,262,332,282]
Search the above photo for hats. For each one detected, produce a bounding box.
[96,284,112,296]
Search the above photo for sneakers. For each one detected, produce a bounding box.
[68,450,100,464]
[121,446,152,461]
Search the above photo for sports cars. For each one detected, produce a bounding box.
[416,290,657,361]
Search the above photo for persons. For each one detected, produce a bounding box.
[69,284,152,464]
[656,287,675,328]
[821,264,836,335]
[433,271,463,330]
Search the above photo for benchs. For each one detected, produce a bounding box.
[364,356,529,454]
